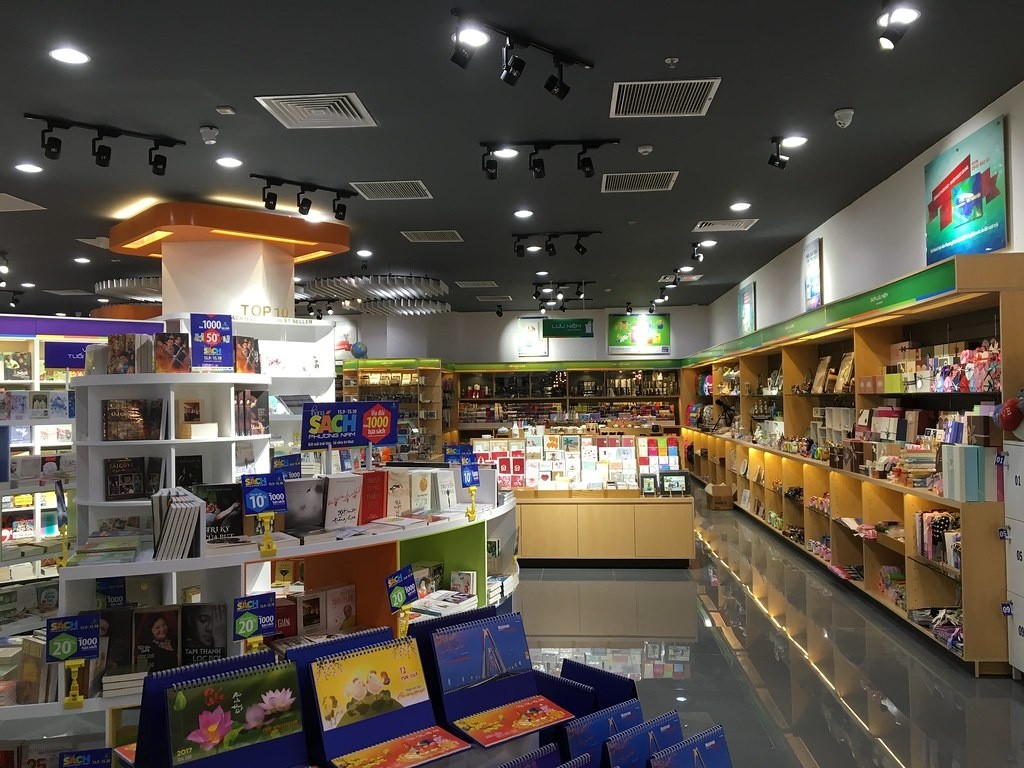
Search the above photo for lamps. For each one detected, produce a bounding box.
[496,305,503,317]
[0,251,25,307]
[514,236,528,257]
[879,0,911,50]
[627,269,681,314]
[297,183,318,215]
[577,144,600,178]
[574,234,587,256]
[482,145,503,179]
[575,284,584,299]
[544,55,570,101]
[533,284,549,314]
[544,234,559,256]
[767,136,790,170]
[692,248,705,262]
[450,19,475,69]
[500,37,527,87]
[528,145,551,178]
[91,127,122,167]
[556,284,569,313]
[306,301,334,320]
[333,192,351,220]
[262,177,284,210]
[41,120,73,160]
[149,138,177,176]
[360,261,368,271]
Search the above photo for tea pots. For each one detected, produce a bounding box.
[498,426,508,433]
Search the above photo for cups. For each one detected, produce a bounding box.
[651,424,662,432]
[482,434,494,438]
[536,425,545,434]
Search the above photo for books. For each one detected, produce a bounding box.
[78,313,515,701]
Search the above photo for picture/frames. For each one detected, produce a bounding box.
[659,470,690,495]
[641,474,657,496]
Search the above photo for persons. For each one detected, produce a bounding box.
[339,605,355,629]
[206,502,240,524]
[144,615,176,671]
[99,618,111,636]
[187,605,226,648]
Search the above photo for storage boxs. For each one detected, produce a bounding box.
[880,417,907,442]
[287,586,325,636]
[905,411,936,443]
[966,416,1003,446]
[262,597,298,644]
[705,482,737,510]
[978,446,1004,501]
[859,341,973,394]
[319,579,355,632]
[941,445,978,502]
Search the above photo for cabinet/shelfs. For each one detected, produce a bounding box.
[153,315,337,475]
[682,252,1024,681]
[1003,440,1024,681]
[335,358,454,463]
[0,372,521,768]
[514,495,695,569]
[0,315,166,639]
[455,359,682,445]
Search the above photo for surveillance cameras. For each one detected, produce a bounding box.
[834,109,855,128]
[200,127,219,145]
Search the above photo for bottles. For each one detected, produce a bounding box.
[511,421,519,438]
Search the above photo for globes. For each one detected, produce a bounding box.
[351,342,367,359]
